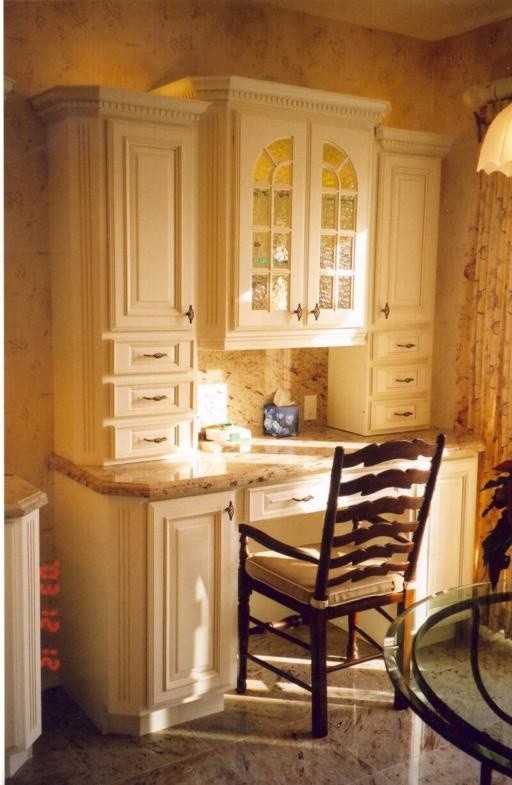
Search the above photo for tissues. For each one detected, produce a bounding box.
[263,390,299,437]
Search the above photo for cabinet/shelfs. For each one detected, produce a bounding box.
[44,424,487,737]
[146,75,393,352]
[30,87,209,465]
[326,126,457,437]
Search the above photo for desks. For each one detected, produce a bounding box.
[383,581,511,785]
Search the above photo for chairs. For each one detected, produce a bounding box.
[239,435,452,739]
